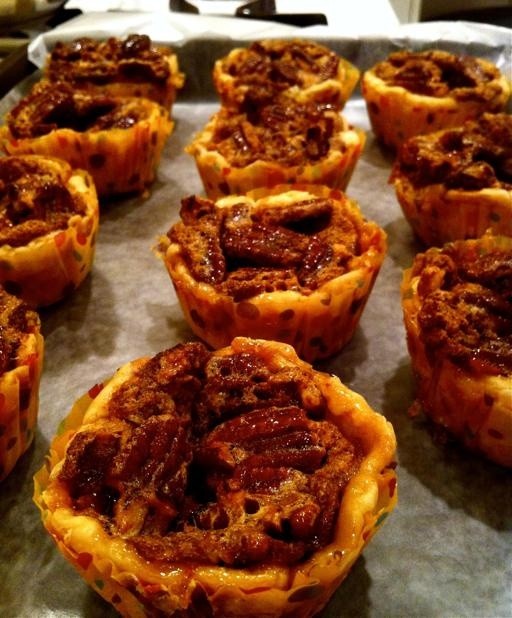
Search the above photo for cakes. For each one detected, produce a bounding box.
[32,334,398,618]
[0,70,175,198]
[0,283,44,486]
[211,38,361,111]
[0,153,100,309]
[400,226,512,470]
[150,183,389,362]
[386,112,512,247]
[186,86,367,202]
[359,48,512,155]
[44,33,187,120]
